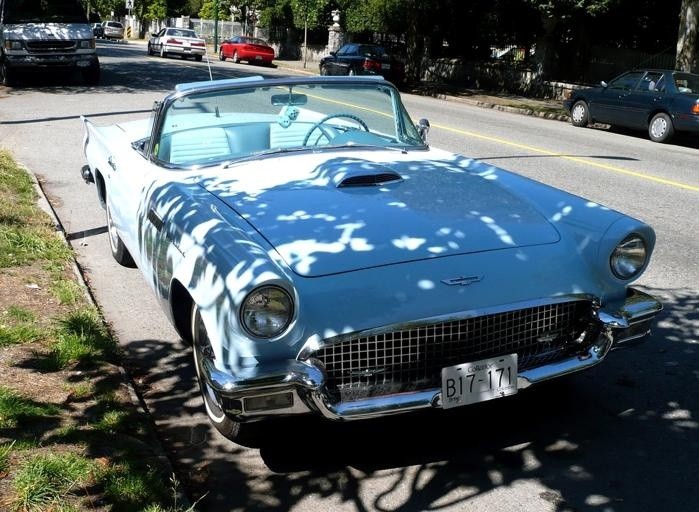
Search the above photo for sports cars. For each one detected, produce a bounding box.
[73,65,677,445]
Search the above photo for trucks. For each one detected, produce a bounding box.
[0,1,105,86]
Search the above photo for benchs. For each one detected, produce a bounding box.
[155,121,345,165]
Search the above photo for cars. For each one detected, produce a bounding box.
[315,40,407,90]
[91,18,209,62]
[560,66,699,148]
[216,34,274,67]
